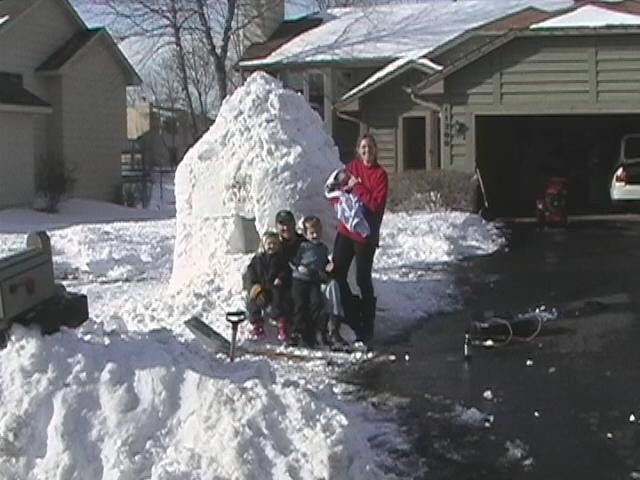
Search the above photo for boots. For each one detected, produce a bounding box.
[278,320,289,340]
[249,322,264,336]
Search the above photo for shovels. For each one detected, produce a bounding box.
[184,317,331,366]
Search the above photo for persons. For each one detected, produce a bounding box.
[239,209,335,351]
[325,133,391,357]
[336,170,351,187]
[241,230,293,342]
[288,215,330,347]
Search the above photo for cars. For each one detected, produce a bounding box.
[608,133,640,213]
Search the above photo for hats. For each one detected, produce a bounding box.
[276,210,292,225]
